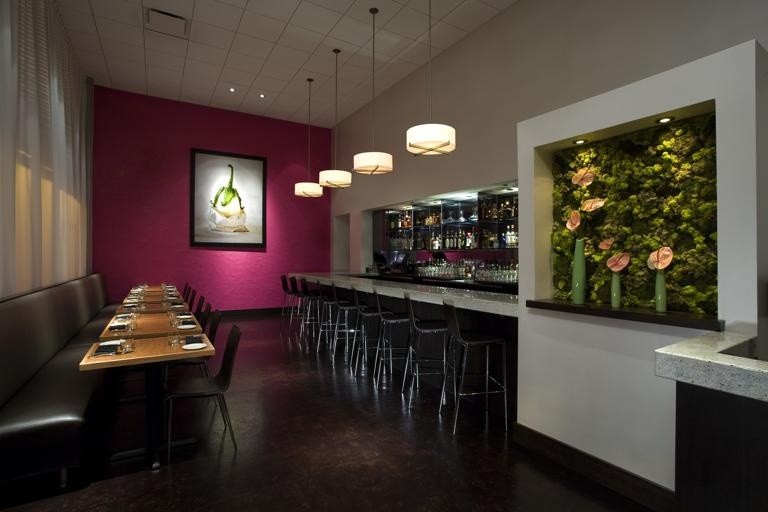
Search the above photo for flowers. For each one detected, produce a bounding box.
[598,238,631,272]
[646,245,674,271]
[565,167,607,239]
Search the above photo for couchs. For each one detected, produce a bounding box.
[1,271,123,509]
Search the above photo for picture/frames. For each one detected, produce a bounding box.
[188,147,268,253]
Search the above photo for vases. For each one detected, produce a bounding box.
[610,271,621,308]
[655,269,669,313]
[571,237,586,304]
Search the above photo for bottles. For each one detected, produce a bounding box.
[368,196,518,289]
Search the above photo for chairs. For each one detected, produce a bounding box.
[280,273,509,440]
[79,282,242,474]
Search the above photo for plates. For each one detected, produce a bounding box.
[94,279,207,357]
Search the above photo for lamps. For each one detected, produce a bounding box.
[294,78,324,199]
[352,8,394,177]
[406,0,457,159]
[318,48,352,190]
